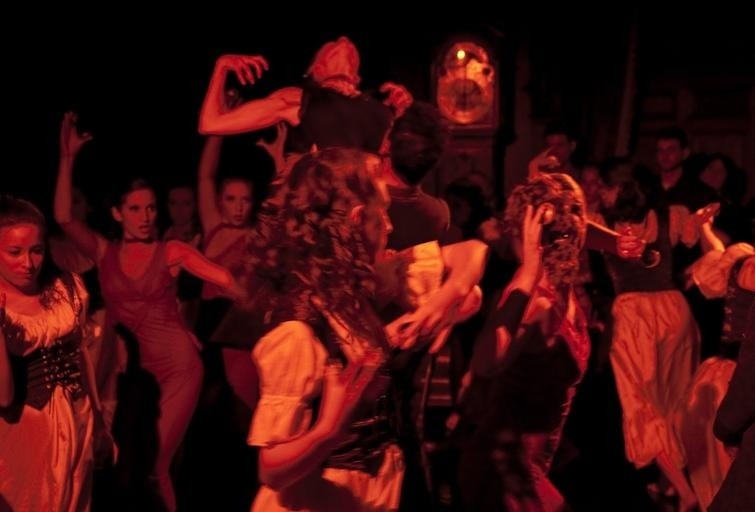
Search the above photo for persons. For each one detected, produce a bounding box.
[3,112,754,511]
[197,36,413,190]
